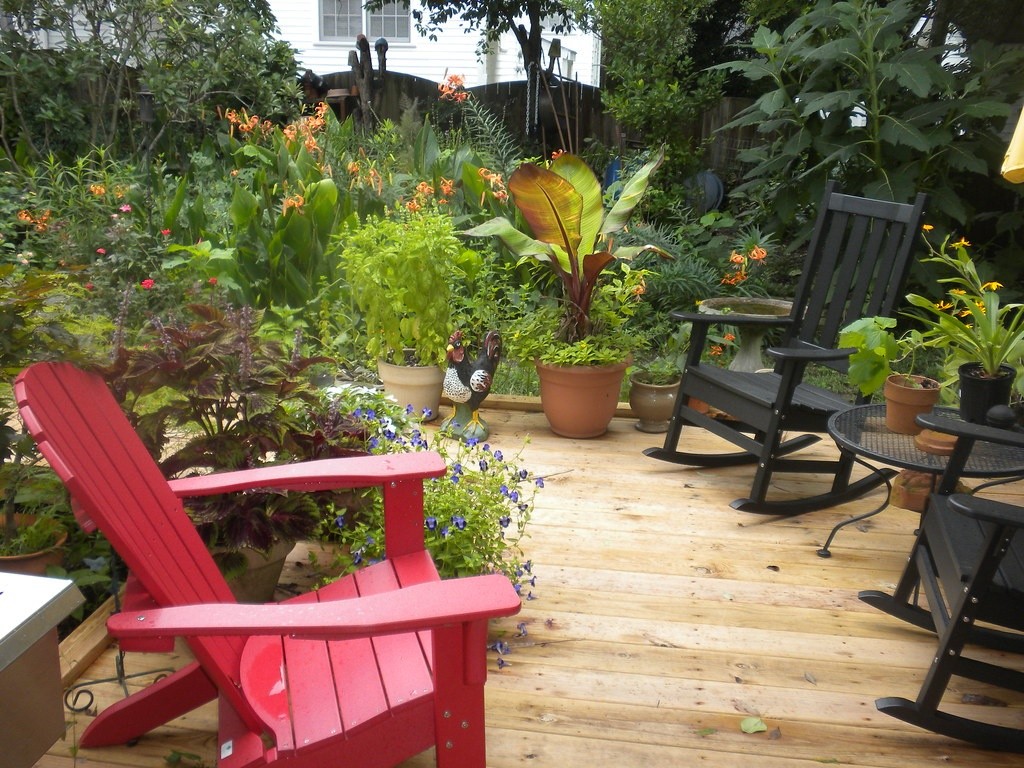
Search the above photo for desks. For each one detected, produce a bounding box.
[817,405,1024,556]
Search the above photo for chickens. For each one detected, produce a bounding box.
[438,329,503,435]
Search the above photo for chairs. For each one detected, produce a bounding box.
[857,414,1024,754]
[640,179,930,515]
[13,360,523,768]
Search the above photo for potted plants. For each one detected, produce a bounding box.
[153,302,343,604]
[0,258,92,574]
[451,145,667,442]
[628,331,691,433]
[838,316,941,436]
[325,213,465,422]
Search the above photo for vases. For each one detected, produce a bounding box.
[700,296,808,375]
[958,361,1017,424]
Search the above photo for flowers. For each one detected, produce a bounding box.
[349,411,546,668]
[897,222,1024,375]
